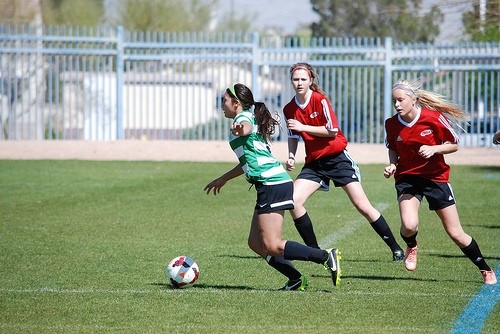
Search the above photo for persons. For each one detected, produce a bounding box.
[282,62,406,265]
[202,83,343,292]
[382,79,498,286]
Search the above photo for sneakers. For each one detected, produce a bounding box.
[480,269,497,285]
[404,241,418,271]
[393,250,405,261]
[323,248,341,286]
[278,275,308,291]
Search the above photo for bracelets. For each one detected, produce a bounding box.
[289,157,294,160]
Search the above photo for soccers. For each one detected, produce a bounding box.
[167,256,199,289]
